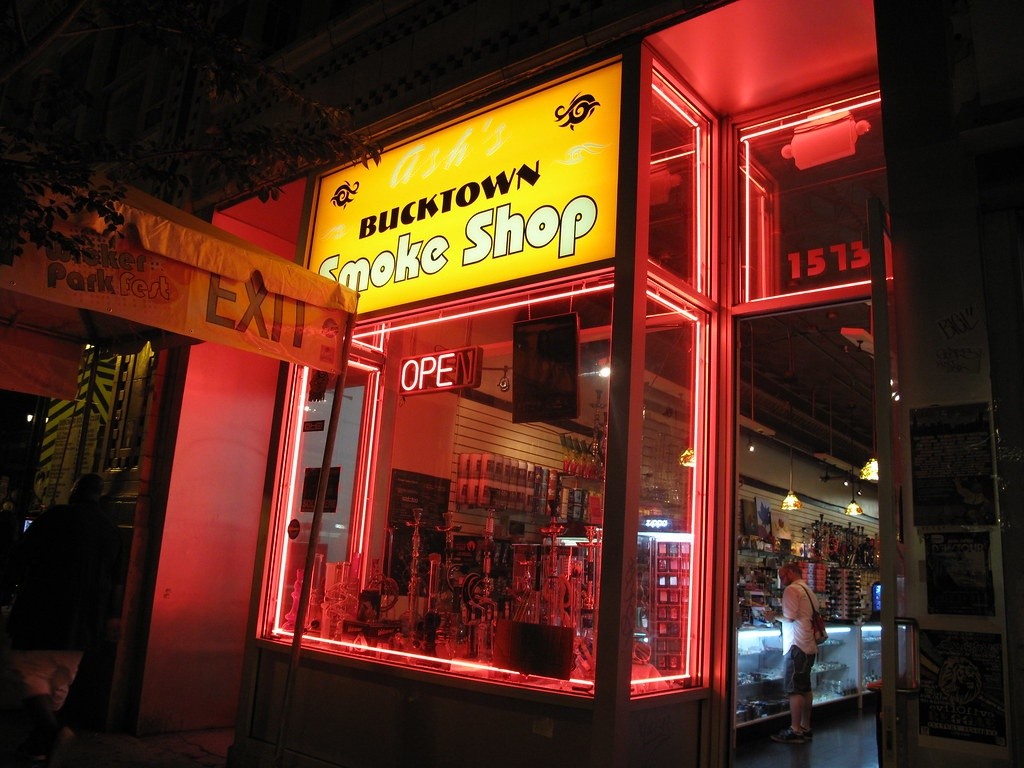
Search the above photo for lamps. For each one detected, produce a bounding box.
[859,355,880,484]
[780,402,803,514]
[845,431,863,517]
[857,489,864,496]
[746,434,756,452]
[677,323,694,468]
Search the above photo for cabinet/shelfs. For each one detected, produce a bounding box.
[732,622,882,750]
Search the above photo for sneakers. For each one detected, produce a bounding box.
[770,724,812,744]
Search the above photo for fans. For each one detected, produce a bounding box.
[819,466,845,482]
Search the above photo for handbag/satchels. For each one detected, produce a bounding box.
[796,583,828,645]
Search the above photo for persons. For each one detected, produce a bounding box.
[1,469,122,768]
[760,562,820,745]
